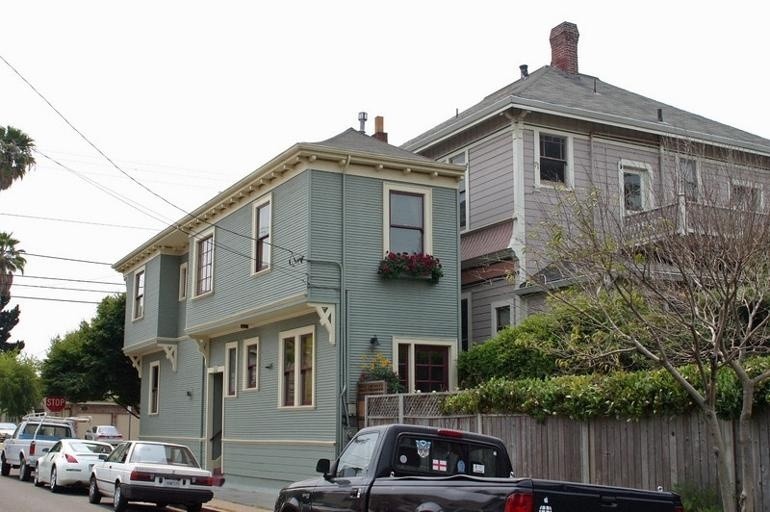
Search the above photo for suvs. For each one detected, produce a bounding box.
[0,412,77,481]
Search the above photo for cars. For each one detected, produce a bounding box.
[84,425,123,445]
[88,439,213,512]
[0,422,17,442]
[34,439,115,492]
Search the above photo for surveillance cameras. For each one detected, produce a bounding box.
[370,337,377,345]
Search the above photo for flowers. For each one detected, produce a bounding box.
[377,250,442,277]
[360,352,393,374]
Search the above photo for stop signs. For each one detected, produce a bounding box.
[46,395,66,412]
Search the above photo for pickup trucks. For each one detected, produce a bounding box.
[273,422,684,512]
